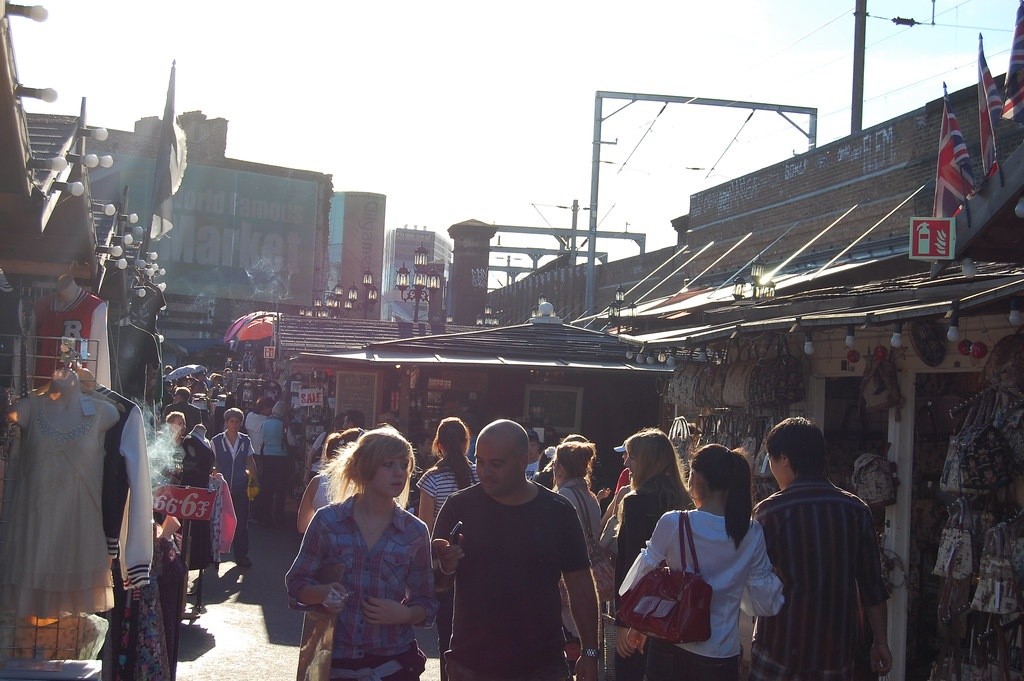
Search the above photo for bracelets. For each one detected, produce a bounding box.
[579,648,601,658]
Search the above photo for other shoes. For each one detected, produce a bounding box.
[239,556,252,567]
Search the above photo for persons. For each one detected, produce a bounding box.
[155,365,692,681]
[285,433,438,681]
[430,419,599,681]
[29,274,111,391]
[618,444,784,681]
[749,417,893,681]
[182,424,215,570]
[0,367,153,617]
[154,514,188,670]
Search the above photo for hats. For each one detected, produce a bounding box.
[614,440,627,452]
[271,401,288,419]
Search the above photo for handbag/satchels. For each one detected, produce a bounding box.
[296,607,335,681]
[666,336,804,504]
[617,511,713,643]
[556,488,619,612]
[928,389,1024,681]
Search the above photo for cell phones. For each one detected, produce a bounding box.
[450,522,463,546]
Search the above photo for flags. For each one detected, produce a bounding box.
[933,92,975,218]
[978,49,1004,176]
[1002,0,1024,125]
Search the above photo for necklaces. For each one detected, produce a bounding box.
[363,514,386,538]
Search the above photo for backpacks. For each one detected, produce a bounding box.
[859,356,900,412]
[852,454,894,508]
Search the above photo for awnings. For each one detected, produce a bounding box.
[620,265,1023,344]
[288,347,678,372]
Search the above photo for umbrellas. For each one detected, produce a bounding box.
[165,365,206,382]
[224,312,281,346]
